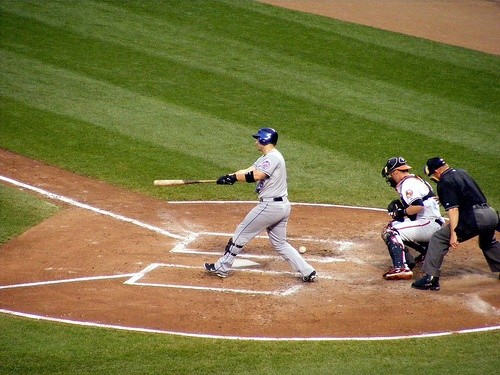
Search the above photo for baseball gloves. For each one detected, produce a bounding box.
[387,199,405,223]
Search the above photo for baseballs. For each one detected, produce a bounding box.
[299,246,306,254]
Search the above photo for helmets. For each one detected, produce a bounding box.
[252,127,278,146]
[424,157,447,176]
[382,157,411,178]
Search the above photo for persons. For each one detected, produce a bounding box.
[380,157,446,280]
[203,128,317,283]
[411,157,500,291]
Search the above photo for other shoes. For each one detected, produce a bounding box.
[303,271,317,282]
[414,248,430,263]
[411,274,440,291]
[204,262,228,277]
[386,268,413,280]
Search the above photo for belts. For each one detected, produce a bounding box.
[435,219,445,227]
[478,203,488,206]
[262,197,283,202]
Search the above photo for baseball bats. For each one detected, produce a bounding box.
[154,180,218,186]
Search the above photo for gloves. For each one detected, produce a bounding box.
[217,173,238,185]
